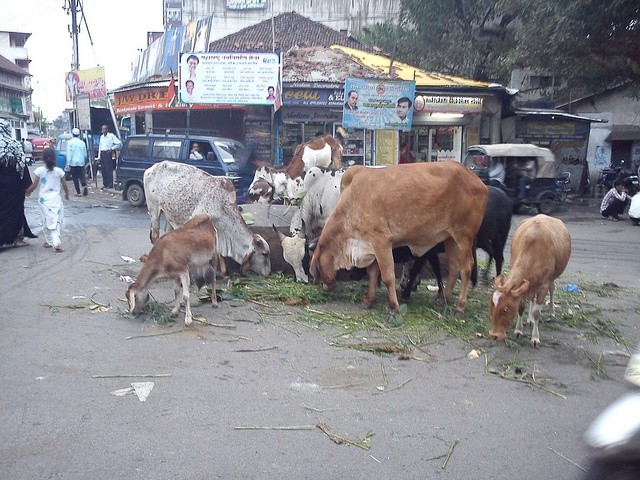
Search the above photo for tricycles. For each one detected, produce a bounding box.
[461,144,561,216]
[55,133,97,180]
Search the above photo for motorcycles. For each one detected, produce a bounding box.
[24,152,36,166]
[601,160,639,197]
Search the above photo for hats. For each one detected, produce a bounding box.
[72,127,80,135]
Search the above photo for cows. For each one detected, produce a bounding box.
[488,214,572,348]
[285,133,342,202]
[350,186,512,300]
[126,215,219,326]
[273,166,357,283]
[309,160,488,327]
[249,165,286,206]
[143,160,271,280]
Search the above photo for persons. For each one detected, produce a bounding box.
[488,157,505,188]
[518,158,537,199]
[184,24,193,52]
[98,125,123,190]
[600,181,632,221]
[400,141,415,163]
[24,139,32,152]
[186,55,199,79]
[25,148,69,252]
[185,80,194,96]
[189,143,206,160]
[266,86,275,100]
[66,128,89,196]
[65,72,80,101]
[0,118,31,246]
[194,19,208,53]
[19,138,24,152]
[345,90,358,110]
[628,191,640,226]
[396,97,412,121]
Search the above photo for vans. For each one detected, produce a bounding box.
[114,128,258,207]
